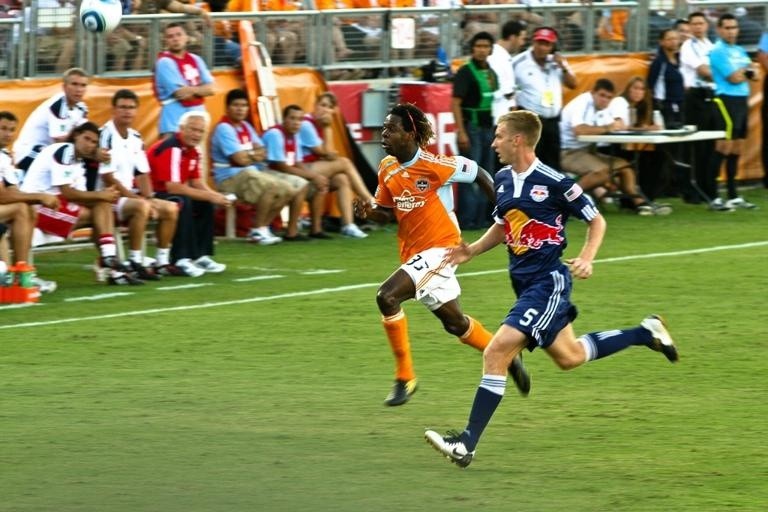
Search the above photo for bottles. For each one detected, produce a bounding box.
[652,110,666,130]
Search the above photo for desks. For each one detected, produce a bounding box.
[579,126,727,217]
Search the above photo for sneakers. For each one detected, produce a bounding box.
[424,430,475,468]
[383,377,418,406]
[637,197,758,217]
[508,351,530,393]
[640,313,678,362]
[248,200,378,245]
[2,254,226,296]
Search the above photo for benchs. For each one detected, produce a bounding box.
[0,194,240,284]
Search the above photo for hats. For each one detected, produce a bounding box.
[532,28,558,43]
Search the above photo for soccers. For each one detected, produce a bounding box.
[79,0,123,34]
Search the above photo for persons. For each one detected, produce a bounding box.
[0,1,767,295]
[423,110,679,467]
[351,101,532,408]
